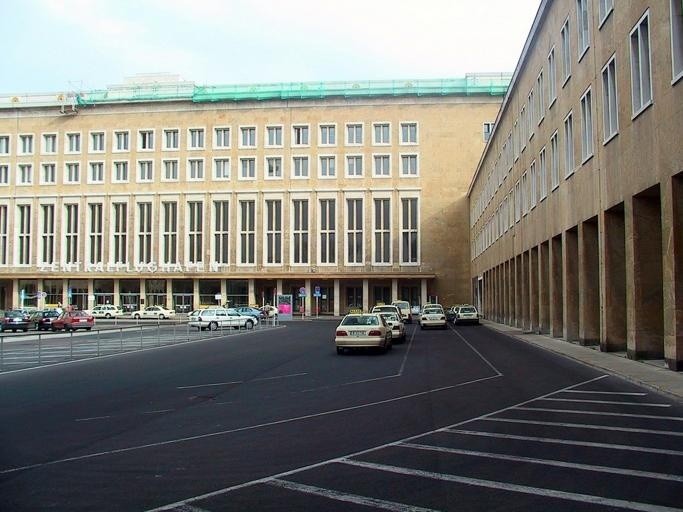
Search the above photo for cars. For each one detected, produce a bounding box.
[234,306,265,325]
[368,298,480,342]
[0,306,95,334]
[333,312,393,354]
[129,304,176,321]
[82,303,124,320]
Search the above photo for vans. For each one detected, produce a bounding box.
[185,308,259,331]
